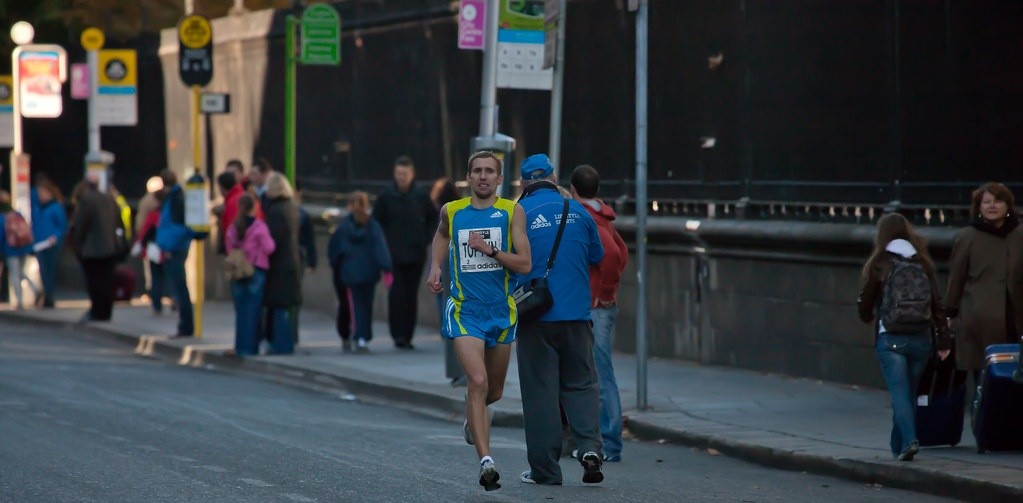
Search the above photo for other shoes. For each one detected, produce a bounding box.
[354,337,369,353]
[395,338,415,351]
[897,444,919,461]
[451,375,467,387]
[343,338,353,354]
[572,448,623,462]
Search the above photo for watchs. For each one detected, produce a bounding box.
[486,246,498,258]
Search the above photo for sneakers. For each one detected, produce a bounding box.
[478,459,501,491]
[521,471,536,483]
[462,391,477,445]
[582,450,605,483]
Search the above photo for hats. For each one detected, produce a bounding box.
[520,154,556,181]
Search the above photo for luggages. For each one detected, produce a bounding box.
[913,330,966,447]
[971,338,1023,454]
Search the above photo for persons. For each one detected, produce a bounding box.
[213,159,317,357]
[857,213,950,461]
[371,156,441,349]
[66,173,131,323]
[132,170,194,339]
[427,151,532,491]
[510,153,606,484]
[0,182,69,311]
[946,184,1023,386]
[328,192,393,352]
[570,165,628,462]
[432,178,466,386]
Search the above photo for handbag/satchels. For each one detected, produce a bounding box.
[511,277,553,320]
[115,258,137,300]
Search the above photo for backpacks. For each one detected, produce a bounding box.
[225,225,259,281]
[880,251,933,330]
[0,210,34,247]
